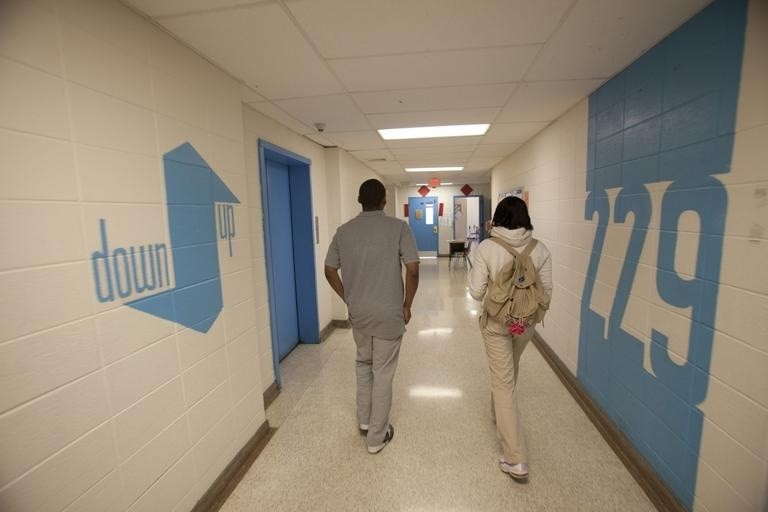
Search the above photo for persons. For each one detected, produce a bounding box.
[324,179,420,453]
[468,197,552,480]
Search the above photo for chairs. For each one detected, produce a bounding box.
[454,241,473,269]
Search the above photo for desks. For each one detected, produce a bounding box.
[448,241,465,270]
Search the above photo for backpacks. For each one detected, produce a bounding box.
[483,237,549,335]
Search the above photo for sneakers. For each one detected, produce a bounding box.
[359,424,394,454]
[498,455,529,479]
